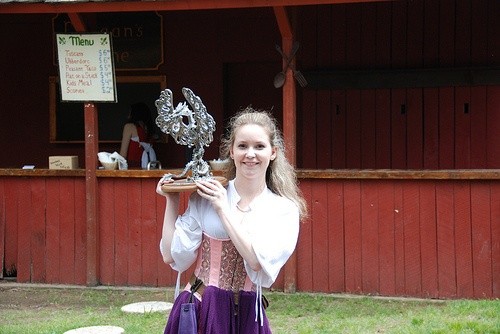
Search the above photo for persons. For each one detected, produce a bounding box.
[160,111,309,334]
[120,102,158,169]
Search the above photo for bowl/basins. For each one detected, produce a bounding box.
[209,161,226,170]
[99,161,118,170]
